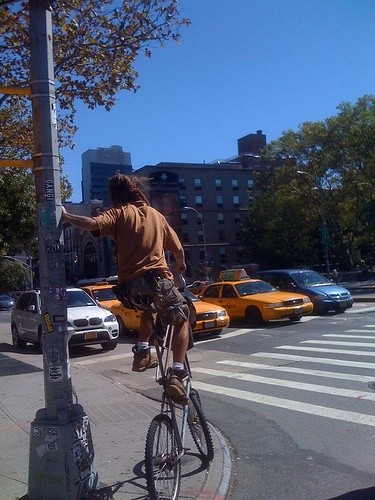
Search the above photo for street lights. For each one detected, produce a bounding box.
[183,206,208,277]
[297,170,330,280]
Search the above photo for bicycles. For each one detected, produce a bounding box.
[128,288,215,500]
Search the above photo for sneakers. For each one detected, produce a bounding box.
[131,344,158,372]
[165,367,190,406]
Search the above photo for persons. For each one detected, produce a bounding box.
[62,171,191,407]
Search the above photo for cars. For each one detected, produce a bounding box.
[0,290,24,311]
[186,277,215,295]
[79,282,121,311]
[10,287,119,351]
[110,286,230,339]
[249,269,354,315]
[194,269,314,328]
